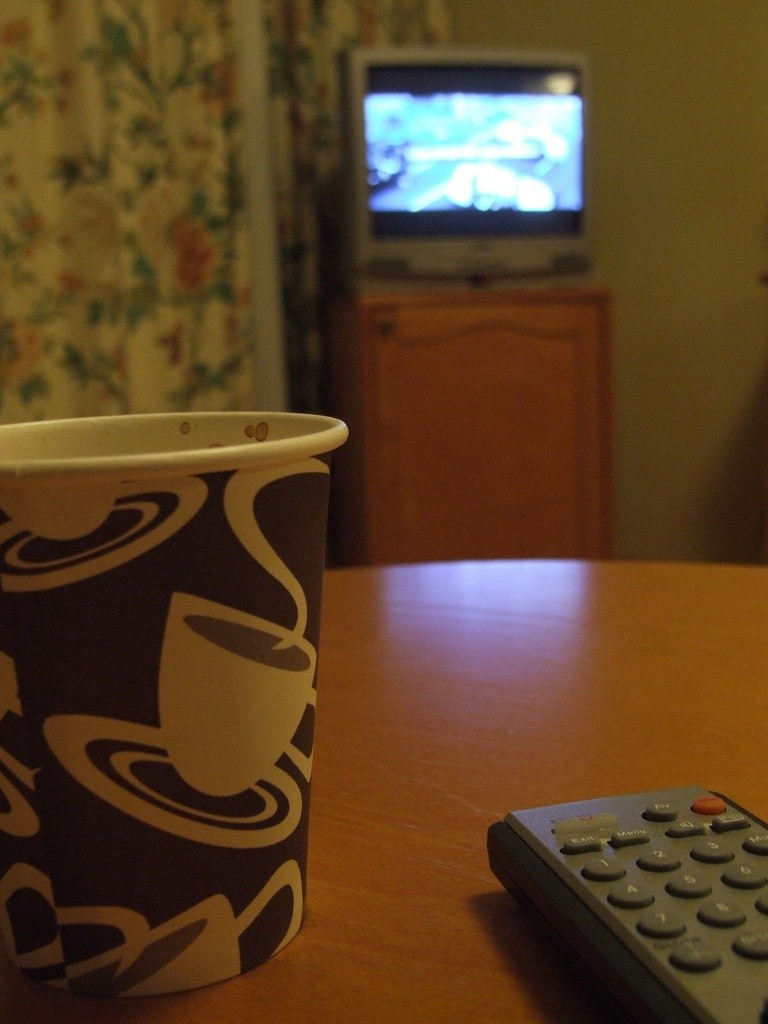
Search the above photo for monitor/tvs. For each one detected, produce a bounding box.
[337,48,592,272]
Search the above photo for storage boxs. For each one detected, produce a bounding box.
[1,412,354,1003]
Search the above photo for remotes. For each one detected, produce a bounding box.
[488,785,767,1023]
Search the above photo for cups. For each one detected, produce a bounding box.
[2,408,352,995]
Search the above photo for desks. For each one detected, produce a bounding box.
[93,553,766,1021]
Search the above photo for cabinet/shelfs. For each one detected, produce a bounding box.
[332,269,623,564]
